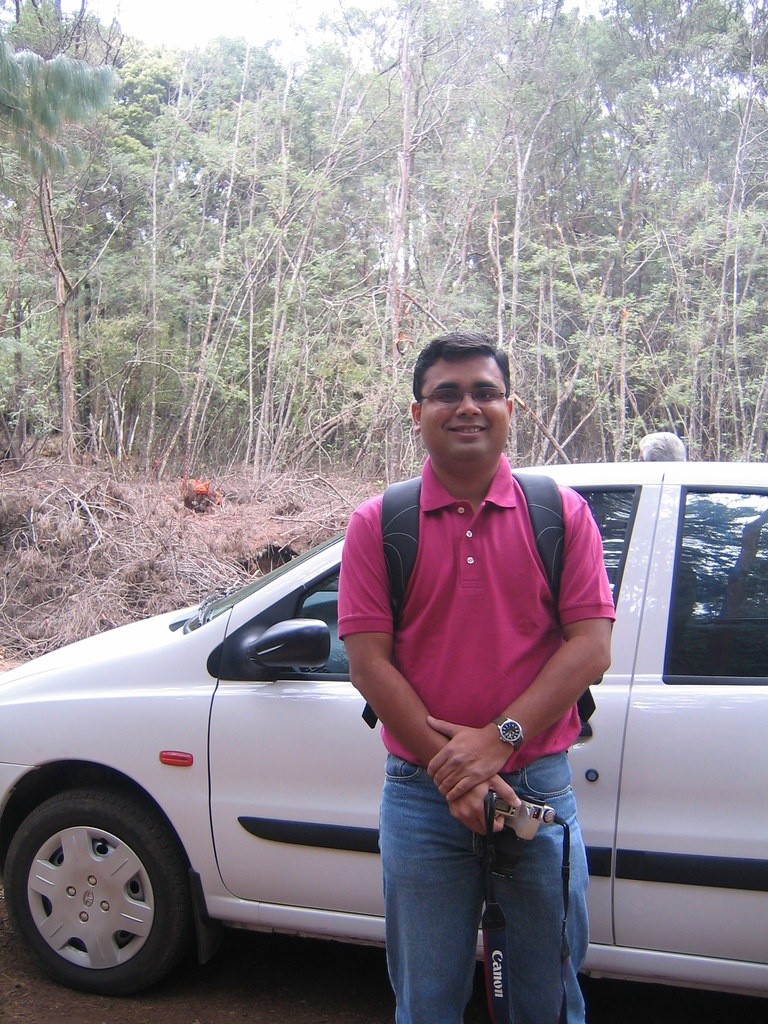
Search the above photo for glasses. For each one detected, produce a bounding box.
[420,387,506,408]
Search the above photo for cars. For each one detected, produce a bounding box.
[0,460,767,1000]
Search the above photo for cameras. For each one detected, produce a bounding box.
[491,793,544,840]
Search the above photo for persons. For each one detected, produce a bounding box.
[336,331,619,1024]
[666,559,713,676]
[636,432,688,462]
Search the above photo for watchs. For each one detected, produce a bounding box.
[491,714,526,750]
